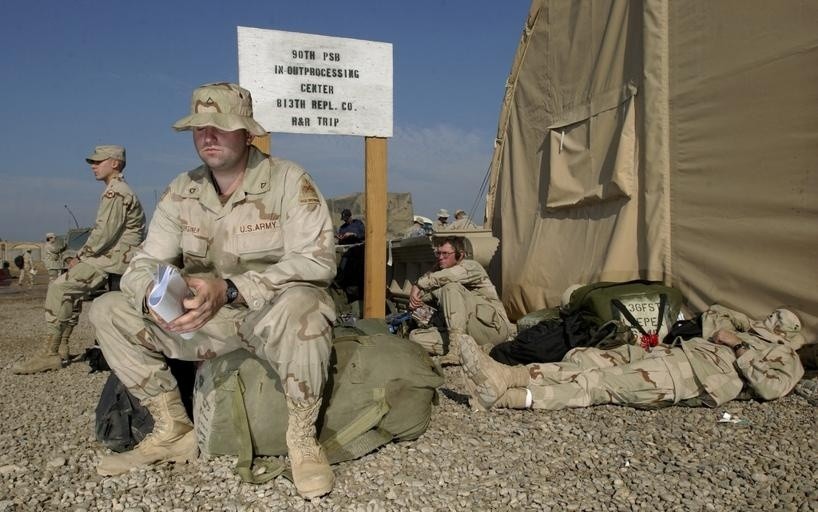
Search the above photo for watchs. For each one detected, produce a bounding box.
[223,278,238,305]
[733,341,749,352]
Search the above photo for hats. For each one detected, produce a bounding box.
[413,217,425,226]
[46,233,57,237]
[435,209,450,218]
[454,208,467,218]
[340,209,352,220]
[748,307,806,350]
[84,144,126,164]
[26,248,32,252]
[171,81,270,140]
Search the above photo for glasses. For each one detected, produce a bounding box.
[434,249,457,258]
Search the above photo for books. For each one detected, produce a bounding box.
[148,262,201,341]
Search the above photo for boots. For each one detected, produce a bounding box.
[10,335,62,374]
[95,385,200,478]
[436,326,465,365]
[285,395,337,499]
[455,334,532,409]
[460,367,527,412]
[57,324,74,359]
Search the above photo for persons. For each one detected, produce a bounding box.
[336,208,365,245]
[18,233,79,287]
[457,304,804,411]
[89,81,338,499]
[12,145,147,375]
[432,209,478,230]
[404,217,426,239]
[408,236,512,366]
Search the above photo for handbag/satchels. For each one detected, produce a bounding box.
[571,276,684,350]
[517,304,569,329]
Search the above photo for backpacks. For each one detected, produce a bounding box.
[189,325,446,484]
[664,312,702,345]
[487,320,573,367]
[93,370,155,451]
[15,255,24,269]
